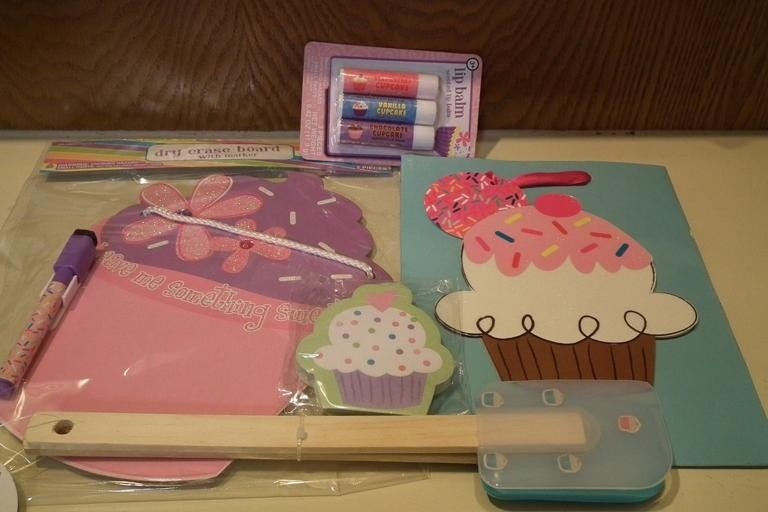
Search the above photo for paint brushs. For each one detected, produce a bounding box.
[338,92,437,126]
[339,68,439,100]
[335,118,435,151]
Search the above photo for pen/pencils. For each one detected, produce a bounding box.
[1,229,98,400]
